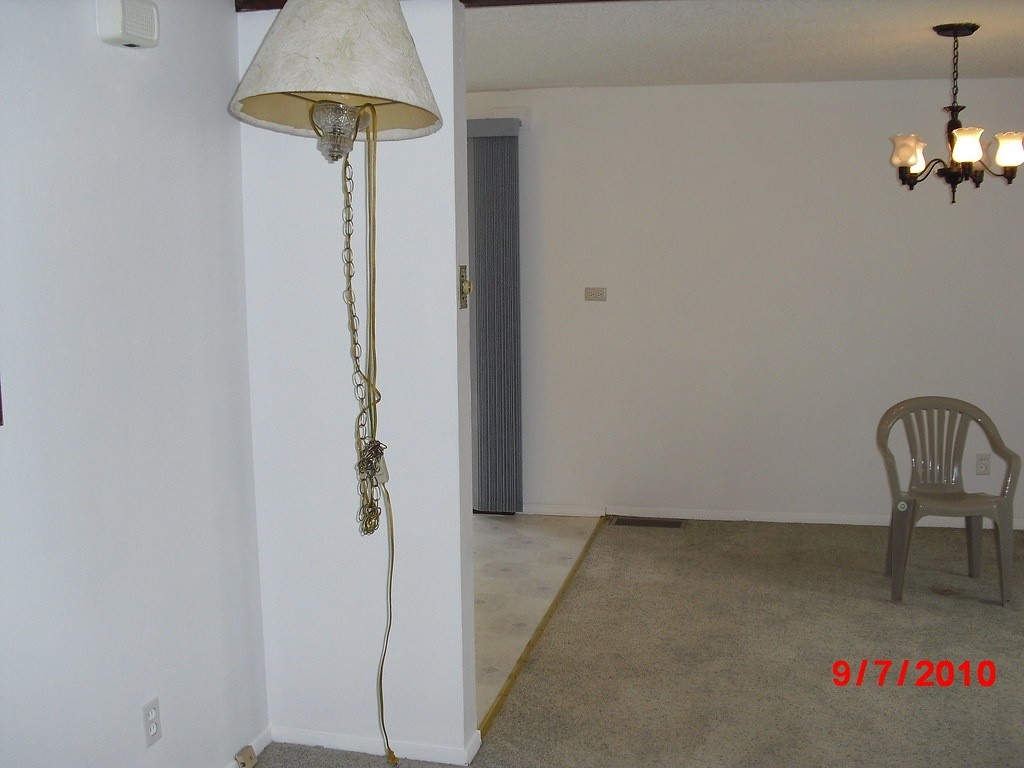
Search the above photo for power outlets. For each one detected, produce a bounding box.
[585,288,606,301]
[976,452,990,475]
[142,698,162,749]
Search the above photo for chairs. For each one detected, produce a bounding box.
[876,396,1021,606]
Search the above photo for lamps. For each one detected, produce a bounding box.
[890,22,1024,204]
[228,0,445,533]
